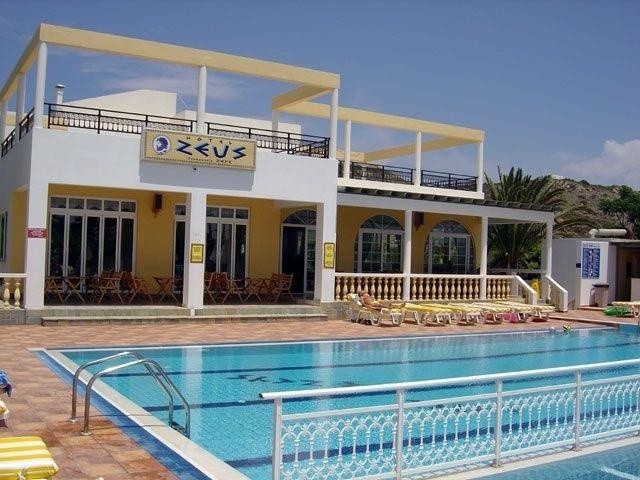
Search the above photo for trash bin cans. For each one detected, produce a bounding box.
[591,282,610,306]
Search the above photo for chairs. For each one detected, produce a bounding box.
[612,301,640,317]
[46,270,294,305]
[347,292,556,325]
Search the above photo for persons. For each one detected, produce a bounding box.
[358,289,406,309]
